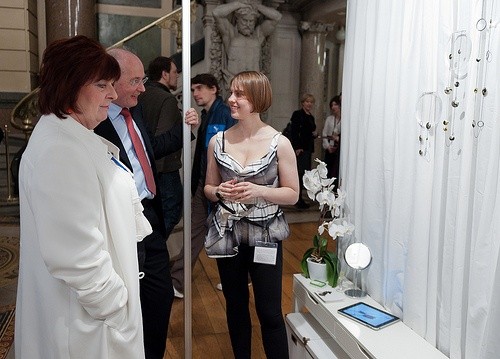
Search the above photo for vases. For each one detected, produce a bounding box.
[307,257,327,283]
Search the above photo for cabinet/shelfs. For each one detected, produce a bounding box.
[285,273,449,359]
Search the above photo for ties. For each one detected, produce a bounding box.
[119,107,157,196]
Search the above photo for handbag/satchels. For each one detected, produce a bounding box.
[281,122,295,148]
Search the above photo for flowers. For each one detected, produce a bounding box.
[300,158,355,288]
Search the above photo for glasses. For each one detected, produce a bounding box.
[121,77,149,86]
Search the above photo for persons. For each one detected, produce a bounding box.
[92,46,200,359]
[171,73,253,299]
[321,91,342,218]
[203,70,301,359]
[12,34,153,359]
[283,93,319,210]
[132,57,185,245]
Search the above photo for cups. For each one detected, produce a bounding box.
[232,178,244,203]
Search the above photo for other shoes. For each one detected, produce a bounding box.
[215,284,222,290]
[172,285,184,299]
[292,199,312,210]
